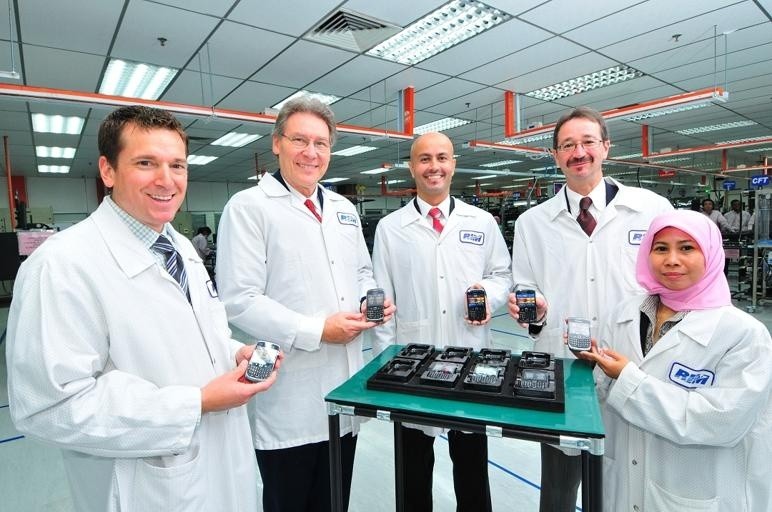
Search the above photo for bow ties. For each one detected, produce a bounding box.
[303,198,323,224]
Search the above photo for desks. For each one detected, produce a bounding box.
[323,345,606,512]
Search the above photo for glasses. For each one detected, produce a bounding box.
[280,131,332,153]
[556,138,604,152]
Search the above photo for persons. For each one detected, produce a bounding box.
[371,132,513,512]
[562,209,772,511]
[191,226,212,259]
[214,96,398,511]
[724,199,751,276]
[700,199,735,232]
[507,106,674,512]
[4,105,284,512]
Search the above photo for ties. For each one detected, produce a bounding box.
[575,196,597,238]
[428,206,444,237]
[151,234,192,308]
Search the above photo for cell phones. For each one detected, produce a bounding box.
[467,289,486,321]
[567,318,591,351]
[516,289,537,323]
[245,341,280,383]
[366,289,384,322]
